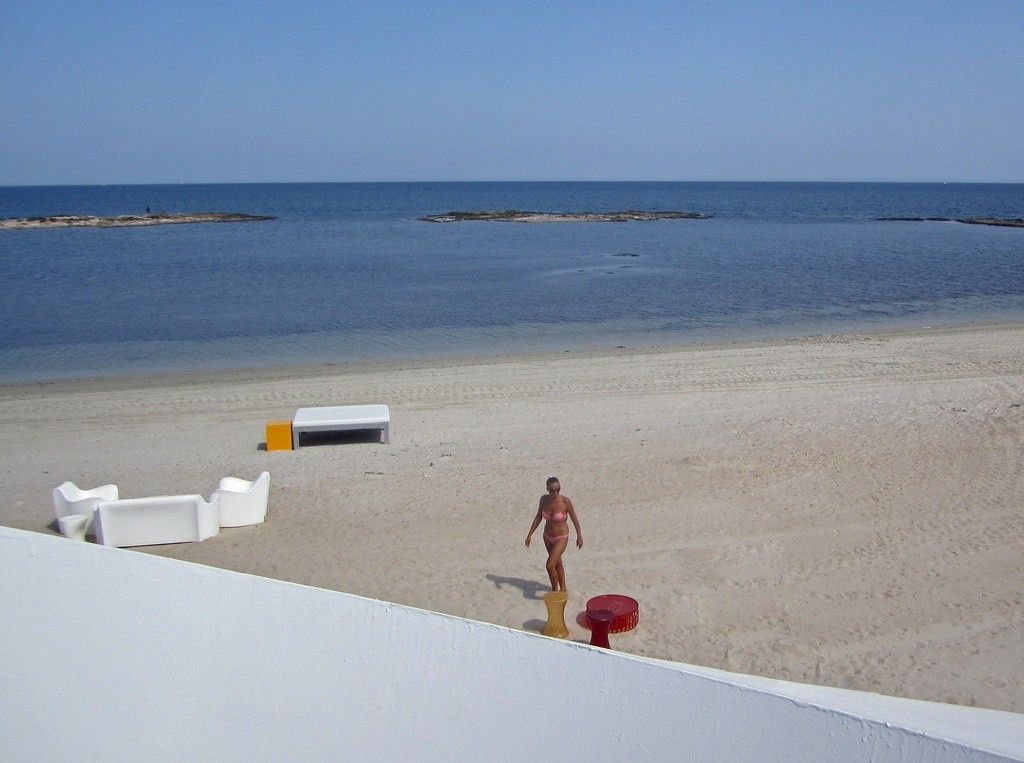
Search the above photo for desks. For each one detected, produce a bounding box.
[264,416,292,452]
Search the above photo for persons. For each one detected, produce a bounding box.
[524,476,584,592]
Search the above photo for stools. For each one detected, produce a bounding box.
[58,513,91,545]
[586,608,612,650]
[541,591,571,640]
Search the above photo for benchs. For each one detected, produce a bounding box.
[291,403,391,450]
[93,492,218,547]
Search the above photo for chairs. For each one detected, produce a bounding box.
[214,470,272,530]
[52,478,118,536]
